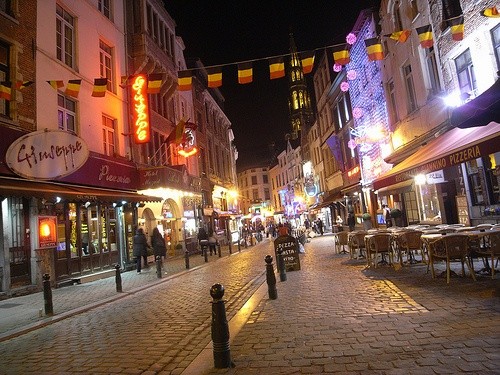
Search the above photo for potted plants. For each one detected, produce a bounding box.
[389,209,402,226]
[363,213,372,229]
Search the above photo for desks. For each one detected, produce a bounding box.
[420,230,498,278]
[364,233,406,267]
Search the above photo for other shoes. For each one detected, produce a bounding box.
[137,272,141,274]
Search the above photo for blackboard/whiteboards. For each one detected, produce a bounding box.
[274,234,300,273]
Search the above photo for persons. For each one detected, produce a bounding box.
[132,227,167,275]
[198,228,218,256]
[336,216,343,232]
[304,218,323,236]
[382,204,393,228]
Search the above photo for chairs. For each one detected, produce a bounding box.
[335,224,500,285]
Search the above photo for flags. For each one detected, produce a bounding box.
[208,67,222,88]
[65,79,81,98]
[147,73,162,93]
[92,78,107,97]
[0,81,12,99]
[47,80,64,89]
[15,81,35,90]
[238,63,253,83]
[301,6,500,74]
[178,70,192,90]
[270,57,284,80]
[165,118,192,149]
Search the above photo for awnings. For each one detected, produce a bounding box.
[373,121,500,190]
[314,190,346,209]
[0,176,163,203]
